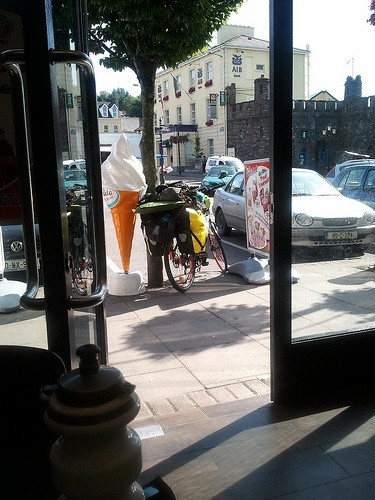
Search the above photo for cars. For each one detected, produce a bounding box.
[325,158,375,211]
[212,167,375,258]
[201,166,236,196]
[0,159,88,287]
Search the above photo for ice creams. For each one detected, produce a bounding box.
[100,132,146,274]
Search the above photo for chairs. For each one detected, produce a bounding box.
[232,187,244,196]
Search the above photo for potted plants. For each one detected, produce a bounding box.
[192,135,206,170]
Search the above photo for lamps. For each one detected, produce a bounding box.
[189,63,200,66]
[207,51,222,57]
[236,49,244,53]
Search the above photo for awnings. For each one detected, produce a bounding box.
[158,124,197,133]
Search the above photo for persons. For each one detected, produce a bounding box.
[202,156,207,173]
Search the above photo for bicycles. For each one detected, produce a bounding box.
[155,182,228,293]
[65,183,98,296]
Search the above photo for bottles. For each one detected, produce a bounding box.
[45,344,146,500]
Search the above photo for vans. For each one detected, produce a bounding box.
[205,156,245,174]
